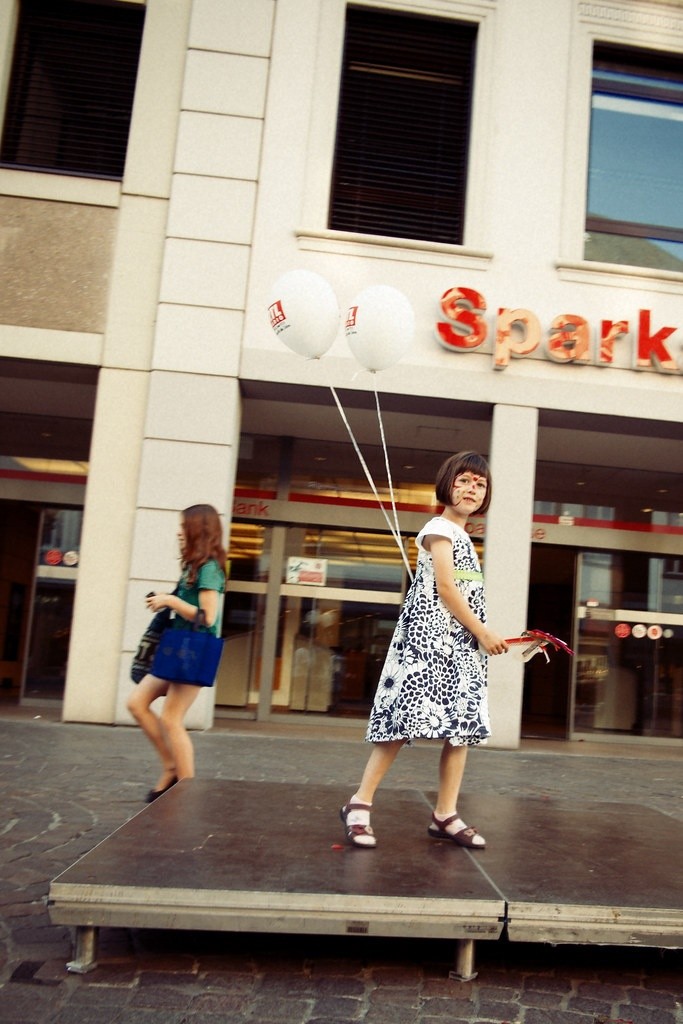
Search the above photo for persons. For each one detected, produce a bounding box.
[126,503,228,802]
[339,451,508,848]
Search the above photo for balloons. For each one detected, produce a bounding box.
[267,270,340,360]
[345,285,415,374]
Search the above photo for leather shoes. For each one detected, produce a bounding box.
[143,772,179,803]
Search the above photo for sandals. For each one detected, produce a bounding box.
[339,802,376,849]
[427,810,487,849]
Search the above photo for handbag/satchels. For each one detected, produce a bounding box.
[150,607,225,690]
[131,611,174,685]
